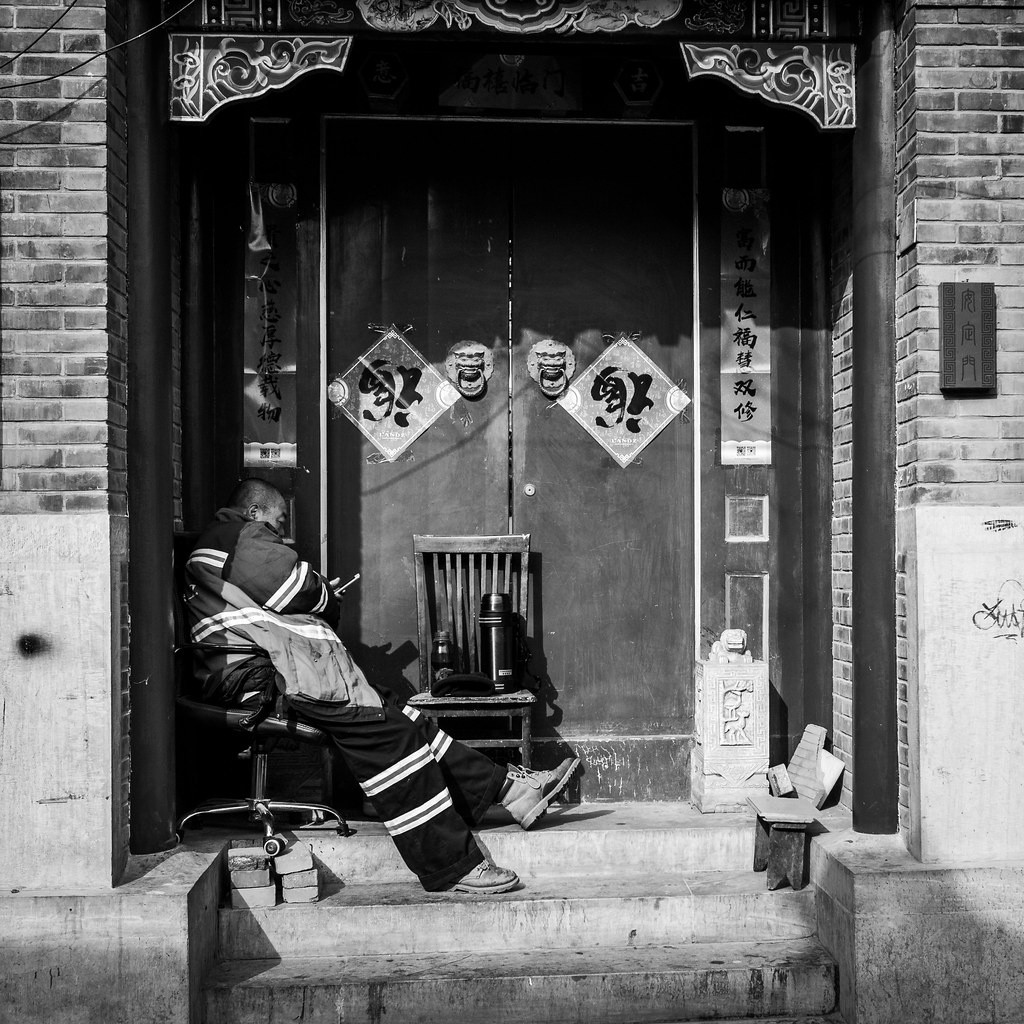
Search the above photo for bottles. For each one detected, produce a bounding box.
[431,631,457,690]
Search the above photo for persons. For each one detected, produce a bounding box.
[177,479,583,898]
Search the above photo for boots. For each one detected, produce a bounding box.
[440,858,520,894]
[496,757,582,830]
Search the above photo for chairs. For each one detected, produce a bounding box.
[173,533,353,856]
[406,533,539,770]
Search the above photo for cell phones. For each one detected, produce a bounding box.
[333,572,360,596]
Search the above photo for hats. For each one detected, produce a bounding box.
[431,671,495,696]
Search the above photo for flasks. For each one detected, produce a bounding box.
[478,593,517,692]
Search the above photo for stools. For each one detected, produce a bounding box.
[746,795,818,893]
